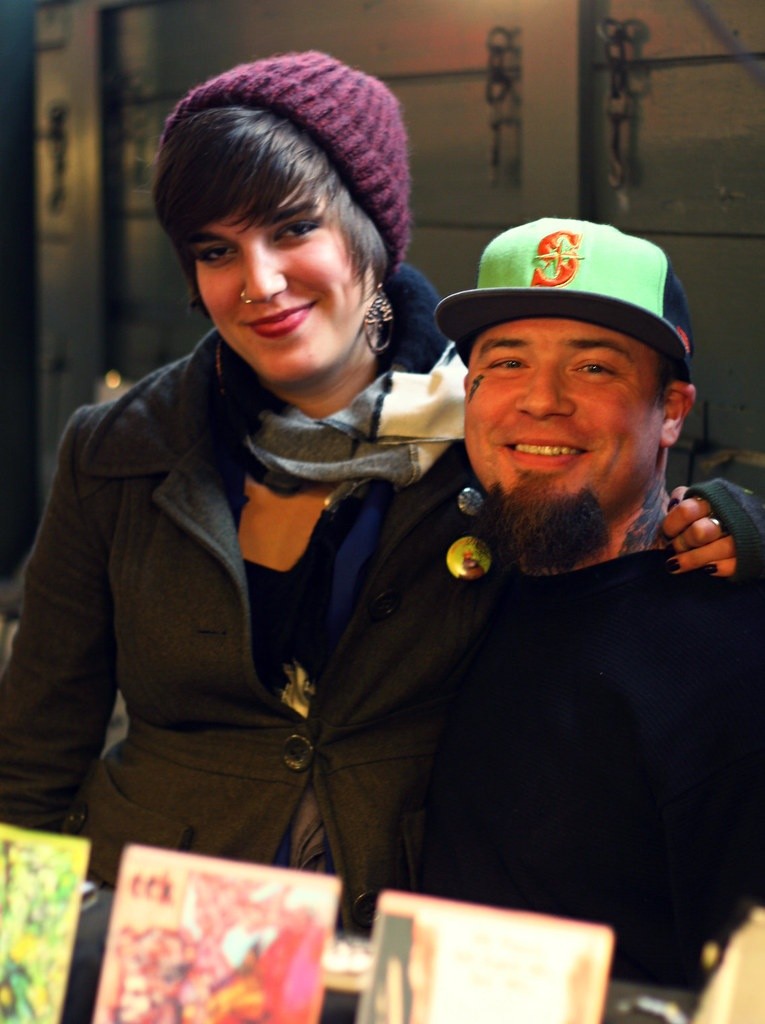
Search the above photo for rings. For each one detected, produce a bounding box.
[694,494,704,503]
[709,516,723,535]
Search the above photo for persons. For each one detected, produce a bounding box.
[0,52,765,996]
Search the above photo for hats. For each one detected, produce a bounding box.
[156,50,412,274]
[434,217,695,384]
